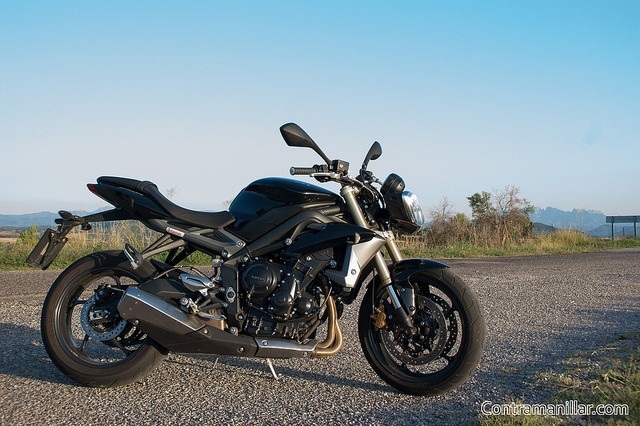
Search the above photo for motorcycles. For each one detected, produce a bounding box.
[26,122,485,396]
